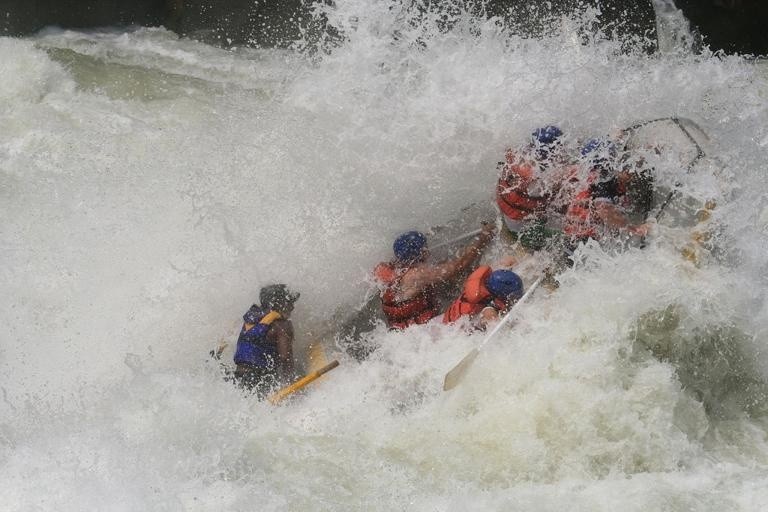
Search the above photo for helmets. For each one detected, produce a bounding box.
[484,269,524,302]
[393,230,427,262]
[260,284,301,313]
[533,126,617,167]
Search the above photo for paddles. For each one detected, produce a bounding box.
[443,253,565,390]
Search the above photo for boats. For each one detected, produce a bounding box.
[216,117,718,394]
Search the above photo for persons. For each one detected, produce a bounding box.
[232,279,309,407]
[557,134,663,252]
[498,121,589,252]
[372,217,494,327]
[434,261,529,334]
